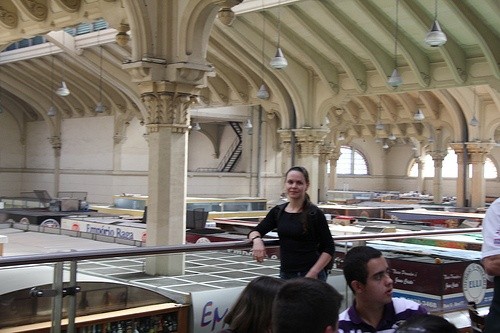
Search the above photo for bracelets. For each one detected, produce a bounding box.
[252,236,261,240]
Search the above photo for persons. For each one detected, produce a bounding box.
[217,276,286,333]
[337,245,427,333]
[481,197,500,333]
[247,166,335,282]
[272,277,344,333]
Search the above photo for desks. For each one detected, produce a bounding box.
[114,188,500,296]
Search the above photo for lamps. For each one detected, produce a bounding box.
[469,87,480,127]
[269,0,288,71]
[387,1,403,87]
[375,95,425,151]
[46,56,56,119]
[255,0,271,100]
[57,51,70,97]
[95,44,106,112]
[424,0,447,49]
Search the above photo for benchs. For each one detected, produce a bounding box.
[21,188,87,212]
